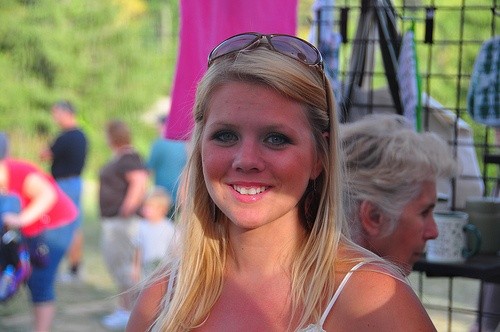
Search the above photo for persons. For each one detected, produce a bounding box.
[99,119,150,327]
[0,157,79,332]
[130,193,175,286]
[336,114,458,278]
[145,116,189,196]
[124,32,437,332]
[40,101,86,282]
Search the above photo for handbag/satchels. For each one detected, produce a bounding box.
[332,5,486,214]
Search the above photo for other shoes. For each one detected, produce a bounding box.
[102,306,130,328]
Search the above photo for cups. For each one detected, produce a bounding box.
[465,197,500,253]
[425,210,481,264]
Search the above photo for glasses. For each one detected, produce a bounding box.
[206,31,327,88]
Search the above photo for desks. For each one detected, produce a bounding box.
[413,252,500,332]
